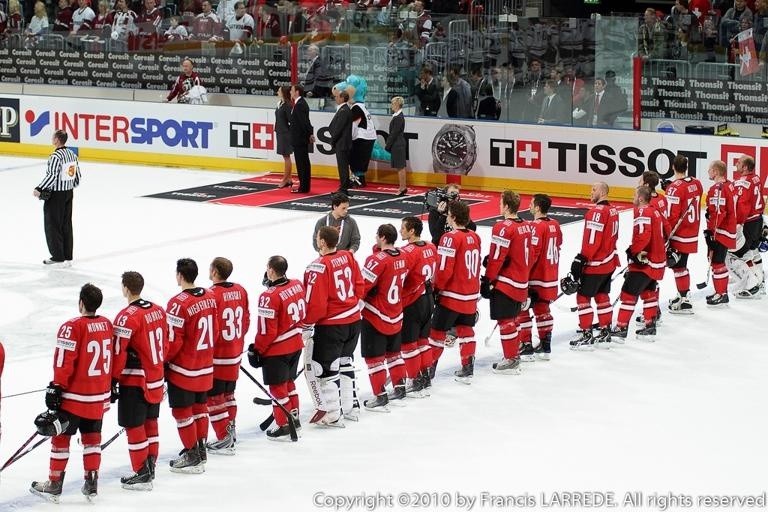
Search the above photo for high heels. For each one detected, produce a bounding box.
[394,189,407,197]
[277,181,290,188]
[289,181,294,187]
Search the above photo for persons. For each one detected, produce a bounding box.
[383,94,407,197]
[1,153,765,497]
[290,82,315,194]
[327,90,352,197]
[1,0,768,126]
[32,129,81,262]
[273,85,294,188]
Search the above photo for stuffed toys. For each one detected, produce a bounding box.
[330,73,391,188]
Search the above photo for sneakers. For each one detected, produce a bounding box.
[267,362,436,437]
[452,309,661,379]
[291,189,310,193]
[65,260,73,265]
[331,189,347,195]
[668,271,768,309]
[32,422,237,496]
[44,258,66,265]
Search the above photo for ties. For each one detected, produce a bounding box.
[546,97,549,109]
[594,93,600,114]
